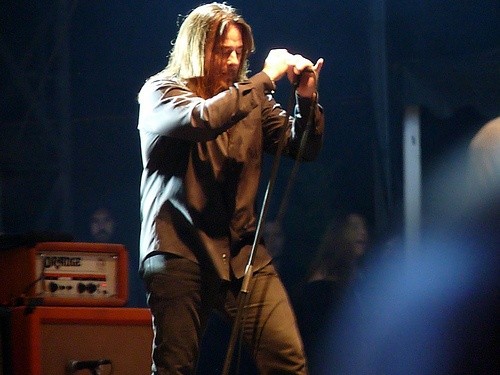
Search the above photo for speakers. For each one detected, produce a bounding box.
[1,242,154,375]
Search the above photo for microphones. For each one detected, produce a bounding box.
[303,67,312,73]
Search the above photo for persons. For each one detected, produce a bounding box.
[252,217,288,260]
[89,204,137,308]
[137,2,317,375]
[306,208,367,314]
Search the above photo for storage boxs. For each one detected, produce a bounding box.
[0,304,153,375]
